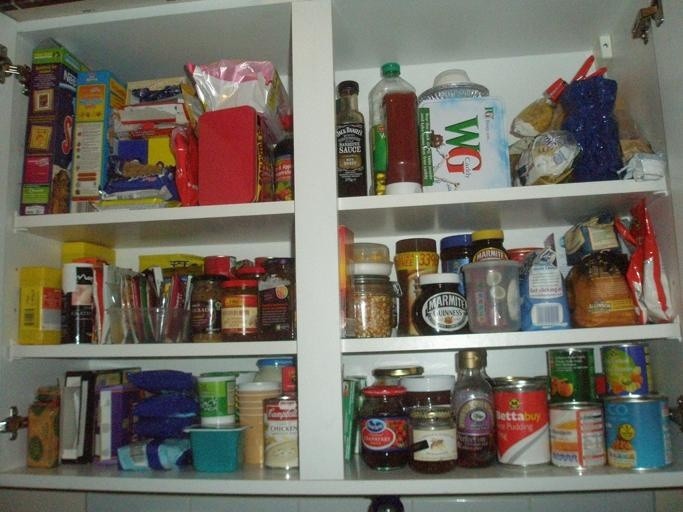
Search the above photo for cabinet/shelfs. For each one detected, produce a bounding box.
[0,0,683,512]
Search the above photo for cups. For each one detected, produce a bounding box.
[195,372,239,427]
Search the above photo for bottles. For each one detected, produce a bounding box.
[370,62,417,195]
[269,113,292,201]
[334,79,365,196]
[346,227,524,340]
[189,255,295,342]
[238,360,296,468]
[361,348,497,476]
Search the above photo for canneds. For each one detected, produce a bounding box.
[347,228,512,339]
[359,385,410,471]
[372,365,456,419]
[549,401,606,470]
[547,347,597,404]
[62,228,296,344]
[264,396,299,469]
[602,393,674,471]
[408,407,458,472]
[492,376,551,467]
[595,343,655,398]
